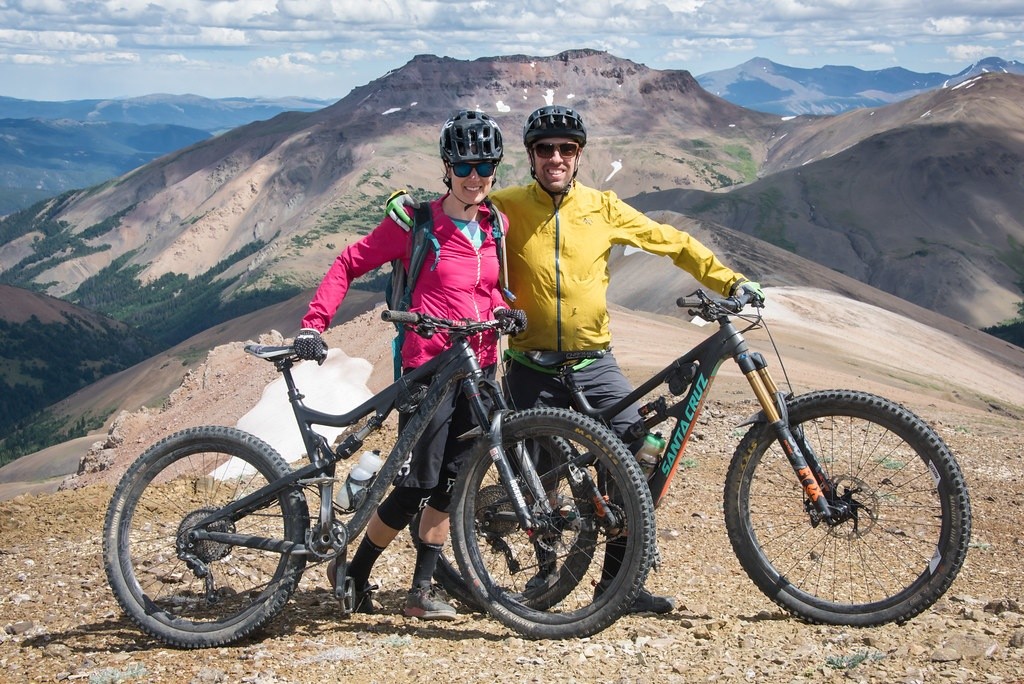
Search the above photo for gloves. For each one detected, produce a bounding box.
[736,282,765,305]
[494,308,527,336]
[294,328,329,366]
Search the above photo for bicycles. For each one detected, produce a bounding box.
[102,309,655,650]
[409,293,972,629]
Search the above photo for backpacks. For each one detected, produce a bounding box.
[385,199,503,380]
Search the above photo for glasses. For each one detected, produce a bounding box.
[447,159,500,176]
[532,143,580,160]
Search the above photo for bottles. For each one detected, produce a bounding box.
[636,431,665,480]
[335,450,382,512]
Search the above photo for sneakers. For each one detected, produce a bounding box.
[593,581,676,614]
[328,560,374,614]
[403,580,458,622]
[525,569,559,595]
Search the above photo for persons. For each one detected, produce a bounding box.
[291,106,510,620]
[381,107,767,618]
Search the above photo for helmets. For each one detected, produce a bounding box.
[440,111,503,171]
[524,105,586,150]
